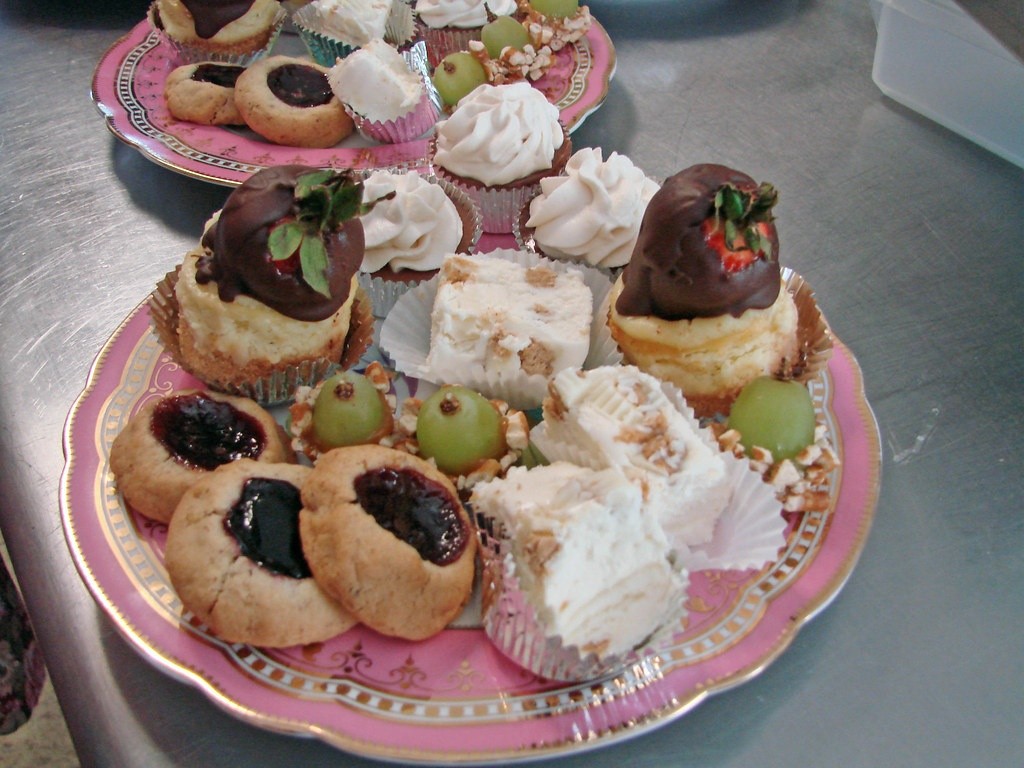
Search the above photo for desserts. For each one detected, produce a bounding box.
[107,0,838,686]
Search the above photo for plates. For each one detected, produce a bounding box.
[91,6,617,189]
[58,232,881,767]
